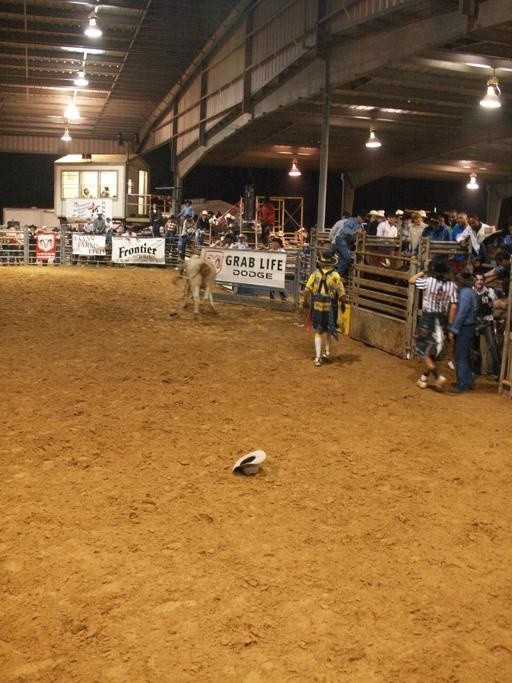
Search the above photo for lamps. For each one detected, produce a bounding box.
[366,123,381,148]
[479,64,502,108]
[60,11,104,142]
[466,167,479,190]
[288,152,302,176]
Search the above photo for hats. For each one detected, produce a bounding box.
[424,211,440,224]
[367,210,385,217]
[428,254,452,273]
[417,210,428,217]
[479,225,503,238]
[356,207,370,221]
[259,196,272,202]
[27,211,112,232]
[455,271,478,283]
[316,251,338,266]
[395,209,404,216]
[231,449,267,476]
[272,238,284,248]
[167,199,236,237]
[236,233,247,242]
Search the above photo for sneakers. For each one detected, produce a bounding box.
[415,379,428,389]
[434,375,448,386]
[448,387,462,393]
[321,352,329,362]
[313,358,321,366]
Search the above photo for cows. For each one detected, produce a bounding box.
[170,254,219,320]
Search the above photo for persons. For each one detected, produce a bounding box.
[298,250,347,367]
[1,186,222,273]
[268,233,291,303]
[223,207,252,295]
[328,208,511,298]
[258,195,275,250]
[446,268,481,392]
[405,259,458,392]
[472,272,501,379]
